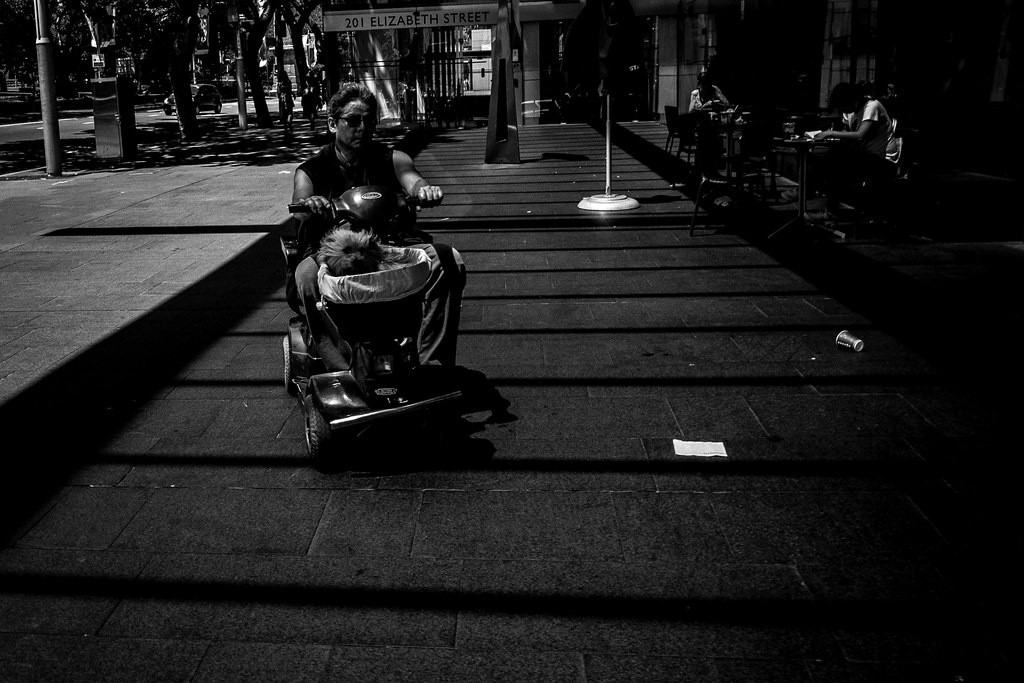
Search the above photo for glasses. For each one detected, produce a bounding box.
[329,116,376,127]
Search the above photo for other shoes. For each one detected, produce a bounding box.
[804,210,838,228]
[781,188,799,201]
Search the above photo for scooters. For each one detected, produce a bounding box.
[278,181,468,470]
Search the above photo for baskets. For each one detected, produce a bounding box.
[316,246,432,338]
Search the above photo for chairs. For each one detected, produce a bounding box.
[662,103,915,239]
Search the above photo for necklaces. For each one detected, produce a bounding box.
[335,145,352,167]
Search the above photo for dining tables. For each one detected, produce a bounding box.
[712,105,752,180]
[765,135,847,240]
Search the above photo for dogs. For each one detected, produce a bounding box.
[318,227,385,276]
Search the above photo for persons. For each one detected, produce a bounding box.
[291,82,466,385]
[781,82,900,221]
[688,72,729,113]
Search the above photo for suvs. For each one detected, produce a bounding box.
[163,82,224,117]
